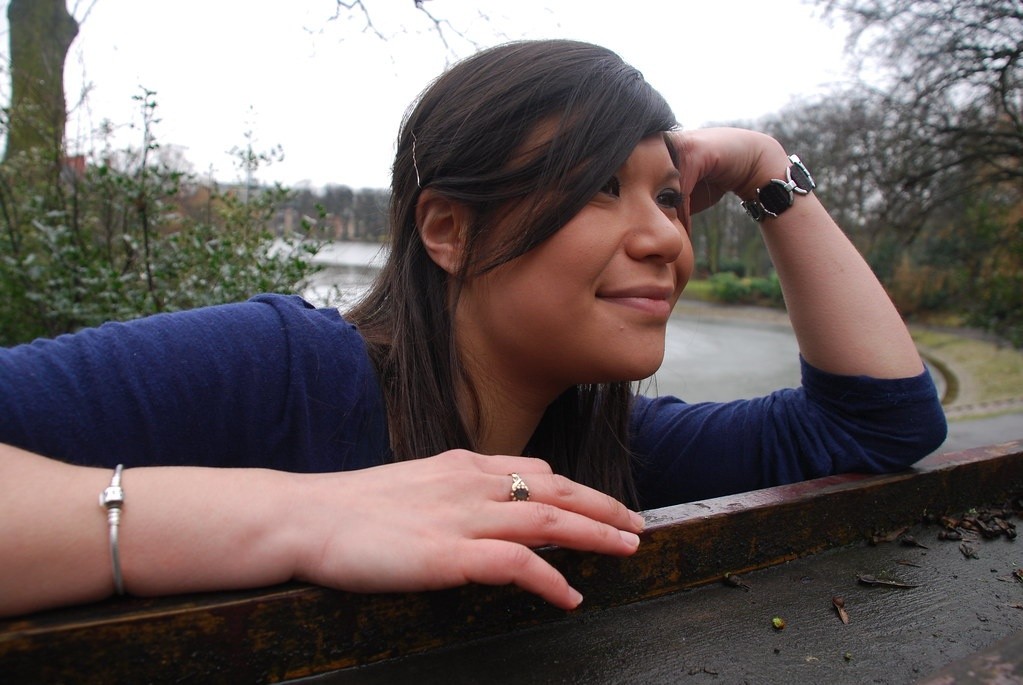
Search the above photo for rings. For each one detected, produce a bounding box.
[506,473,533,505]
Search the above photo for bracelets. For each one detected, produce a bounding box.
[738,153,819,218]
[97,464,140,605]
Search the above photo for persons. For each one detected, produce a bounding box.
[0,37,956,611]
